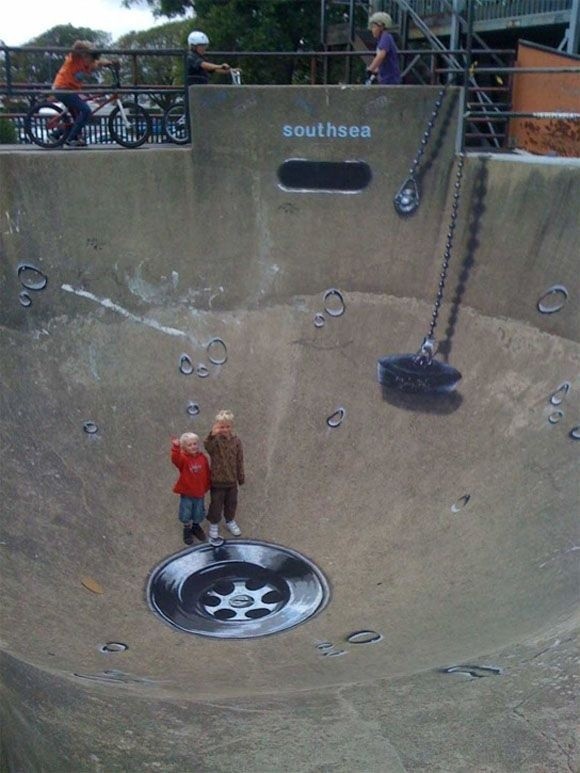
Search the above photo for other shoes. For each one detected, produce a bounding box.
[48,133,59,143]
[66,139,85,146]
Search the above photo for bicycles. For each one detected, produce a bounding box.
[24,58,153,149]
[162,64,242,146]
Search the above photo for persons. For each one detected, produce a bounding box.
[204,408,245,540]
[171,432,210,546]
[184,31,242,146]
[366,11,402,84]
[51,39,112,146]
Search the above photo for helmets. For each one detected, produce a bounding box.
[187,31,210,50]
[368,12,392,30]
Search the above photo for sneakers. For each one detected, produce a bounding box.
[209,521,219,539]
[192,523,206,540]
[225,520,242,536]
[183,528,193,545]
